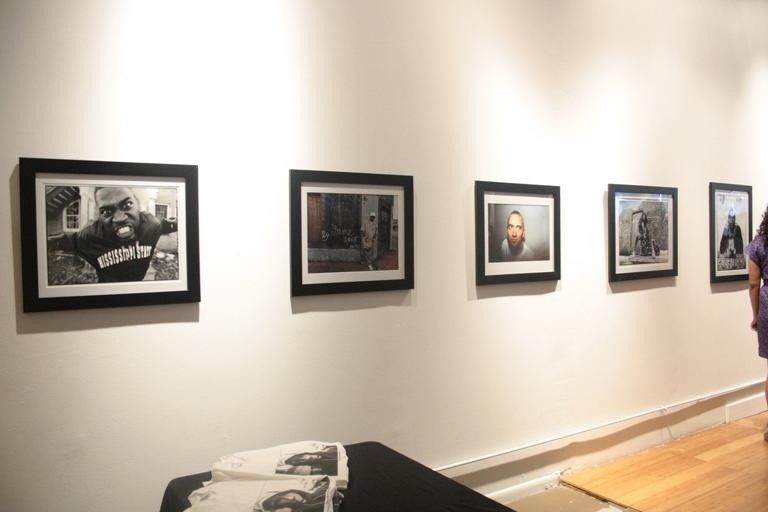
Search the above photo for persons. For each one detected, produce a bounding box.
[501,210,535,261]
[630,213,656,259]
[284,446,337,476]
[359,212,378,271]
[719,208,744,258]
[745,204,768,441]
[47,187,178,283]
[255,480,330,512]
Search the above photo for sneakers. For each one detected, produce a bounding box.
[368,265,373,271]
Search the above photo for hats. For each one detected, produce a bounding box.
[369,212,375,217]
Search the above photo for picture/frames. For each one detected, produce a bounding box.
[288,167,413,298]
[606,181,679,284]
[708,180,755,285]
[472,178,563,287]
[19,157,202,313]
